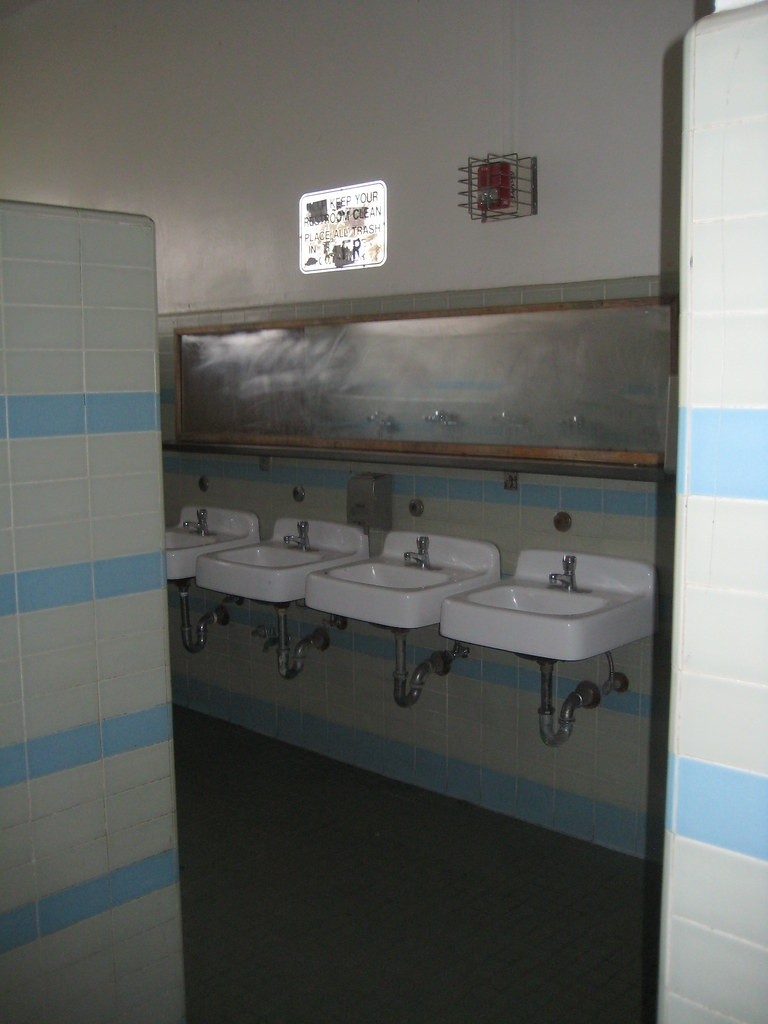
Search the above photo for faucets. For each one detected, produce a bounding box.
[548,554,578,593]
[365,409,397,430]
[422,406,462,427]
[403,535,432,569]
[488,408,526,430]
[556,414,589,433]
[181,508,210,536]
[283,521,311,551]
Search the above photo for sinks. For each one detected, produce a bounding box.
[467,584,608,616]
[164,531,220,549]
[214,545,325,568]
[325,561,452,589]
